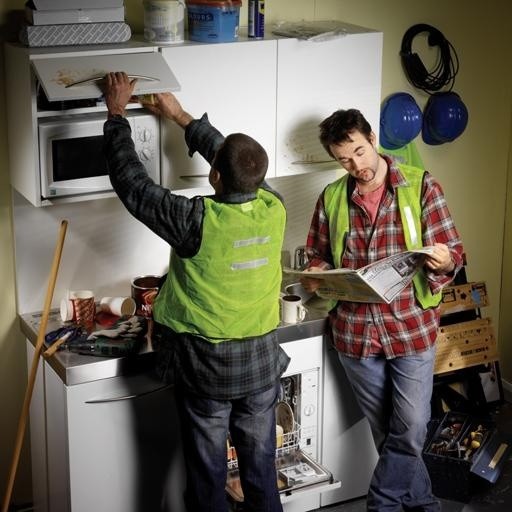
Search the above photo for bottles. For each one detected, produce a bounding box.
[293,246,311,269]
[244,1,266,39]
[276,425,285,448]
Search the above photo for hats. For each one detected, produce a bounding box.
[422,91,470,146]
[381,90,422,150]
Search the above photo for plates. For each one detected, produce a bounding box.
[276,401,296,442]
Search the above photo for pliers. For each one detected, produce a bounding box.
[55,325,81,343]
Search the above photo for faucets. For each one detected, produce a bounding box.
[294,245,306,270]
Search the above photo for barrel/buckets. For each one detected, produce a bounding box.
[186,0,243,43]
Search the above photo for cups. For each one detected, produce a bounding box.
[100,297,136,318]
[282,296,305,325]
[131,274,161,321]
[60,288,97,328]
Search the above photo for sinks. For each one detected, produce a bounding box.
[281,278,312,303]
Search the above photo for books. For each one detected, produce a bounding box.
[283,243,435,305]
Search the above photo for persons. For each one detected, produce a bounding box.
[97,70,291,512]
[298,108,464,511]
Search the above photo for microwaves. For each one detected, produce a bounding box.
[38,114,160,200]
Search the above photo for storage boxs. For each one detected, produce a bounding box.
[420,407,511,502]
